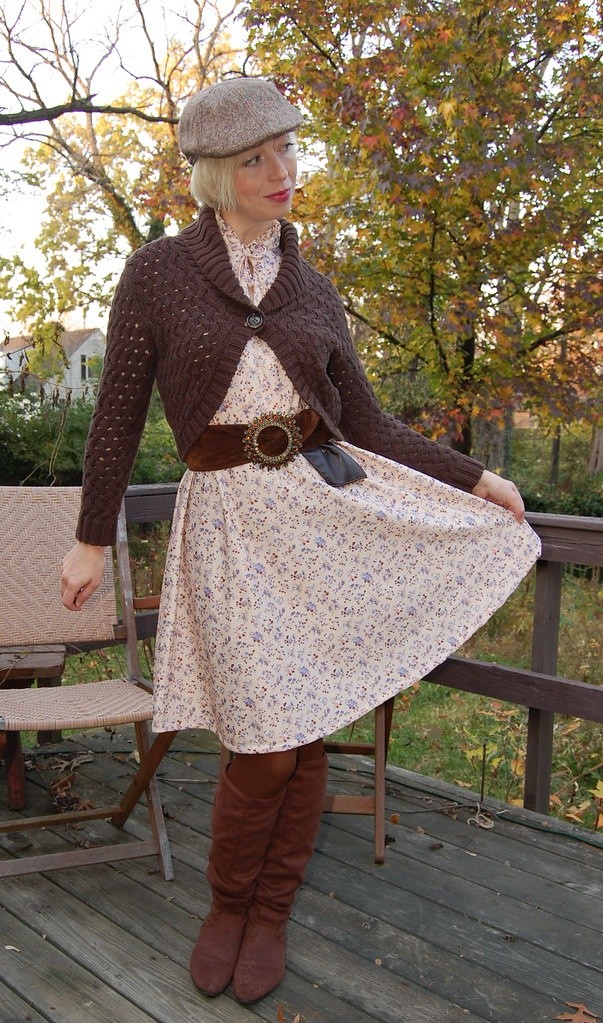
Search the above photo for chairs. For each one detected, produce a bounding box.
[0,485,395,880]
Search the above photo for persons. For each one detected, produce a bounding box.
[60,78,540,1004]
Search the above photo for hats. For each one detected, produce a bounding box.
[177,77,303,158]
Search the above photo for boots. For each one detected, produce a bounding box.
[232,751,329,1004]
[189,760,287,997]
